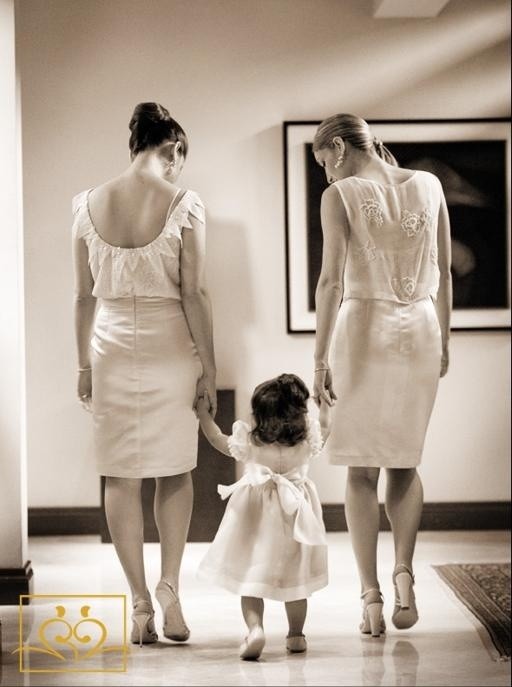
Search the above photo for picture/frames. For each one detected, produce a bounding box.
[282,116,512,335]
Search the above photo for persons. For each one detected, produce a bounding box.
[71,102,218,648]
[196,373,332,659]
[313,113,454,637]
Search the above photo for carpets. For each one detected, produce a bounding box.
[432,562,511,664]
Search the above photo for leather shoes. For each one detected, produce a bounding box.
[285,632,307,652]
[239,624,264,659]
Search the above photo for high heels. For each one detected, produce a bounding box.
[154,576,190,642]
[359,589,385,637]
[391,564,418,629]
[130,600,158,648]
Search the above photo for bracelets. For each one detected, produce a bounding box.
[77,368,92,371]
[314,368,330,371]
